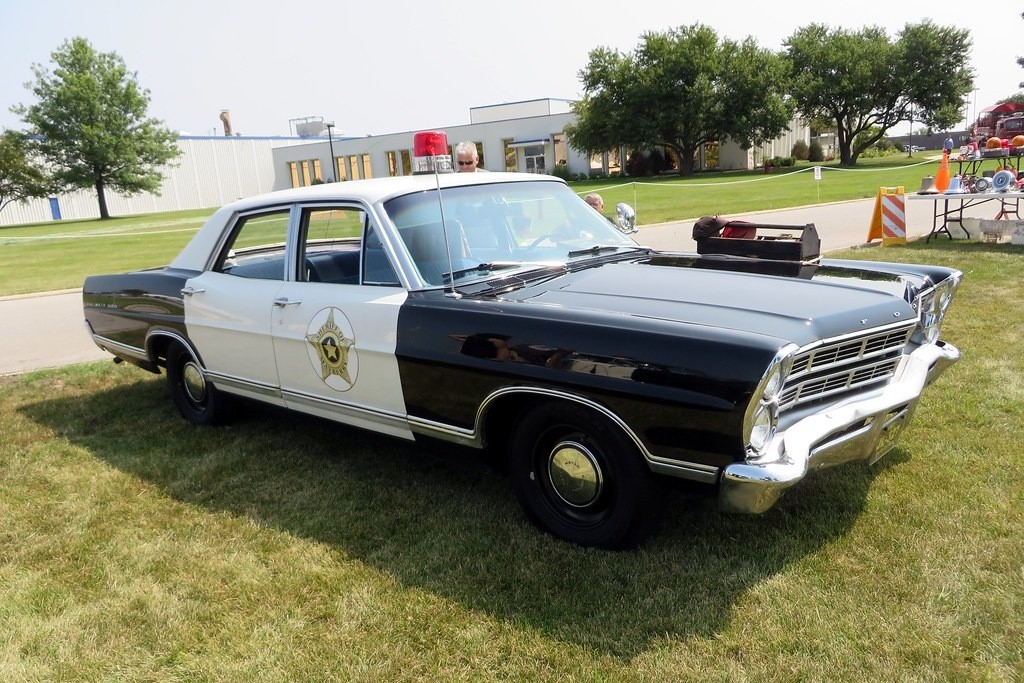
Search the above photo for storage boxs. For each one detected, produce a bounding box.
[978,219,1024,244]
[947,217,983,239]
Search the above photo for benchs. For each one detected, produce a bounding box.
[223,251,332,282]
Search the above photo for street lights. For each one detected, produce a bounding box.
[327,125,337,182]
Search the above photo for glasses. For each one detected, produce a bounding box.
[458,160,473,166]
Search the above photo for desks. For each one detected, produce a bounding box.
[938,157,1024,176]
[908,190,1024,244]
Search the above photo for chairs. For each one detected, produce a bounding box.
[307,250,392,285]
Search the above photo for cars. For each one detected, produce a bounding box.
[84,172,962,546]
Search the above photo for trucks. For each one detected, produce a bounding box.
[976,112,1024,154]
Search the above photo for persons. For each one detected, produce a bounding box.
[551,193,618,247]
[944,134,954,155]
[445,140,501,249]
[965,132,988,149]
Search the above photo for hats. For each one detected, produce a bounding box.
[984,133,988,136]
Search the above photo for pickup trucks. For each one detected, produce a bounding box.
[911,145,925,151]
